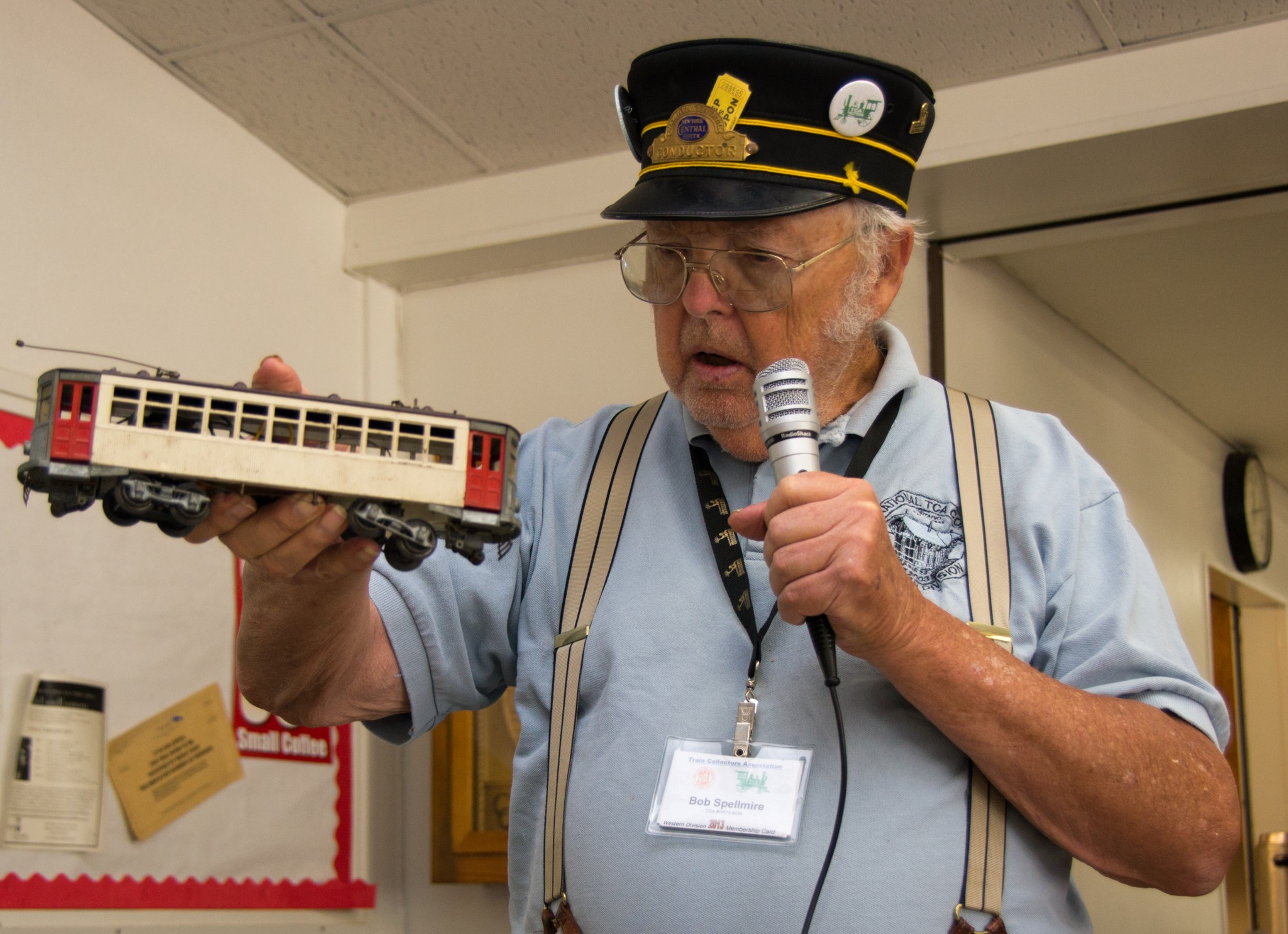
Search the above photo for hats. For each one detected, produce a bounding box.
[598,38,937,223]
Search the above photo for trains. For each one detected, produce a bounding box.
[16,340,520,573]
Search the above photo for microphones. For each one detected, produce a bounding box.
[750,359,841,687]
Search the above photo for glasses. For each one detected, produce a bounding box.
[614,224,884,313]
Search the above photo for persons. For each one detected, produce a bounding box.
[184,33,1244,931]
